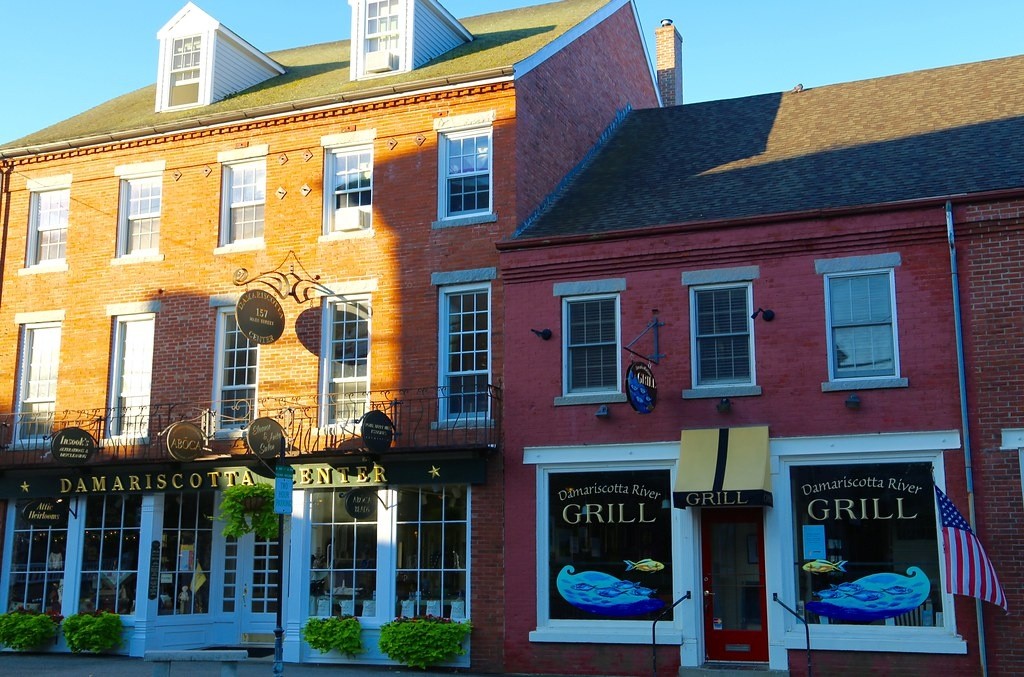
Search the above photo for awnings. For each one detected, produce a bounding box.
[673,424,774,509]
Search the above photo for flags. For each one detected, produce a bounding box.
[934,487,1009,611]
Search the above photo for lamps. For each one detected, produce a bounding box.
[750,308,775,321]
[716,398,735,413]
[594,405,610,420]
[845,394,862,409]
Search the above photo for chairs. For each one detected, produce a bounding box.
[895,599,933,626]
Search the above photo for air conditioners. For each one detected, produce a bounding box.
[365,49,394,72]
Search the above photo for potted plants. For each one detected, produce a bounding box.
[203,483,280,539]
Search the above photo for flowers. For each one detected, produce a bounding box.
[301,614,370,660]
[378,614,475,670]
[62,609,126,655]
[0,604,64,652]
